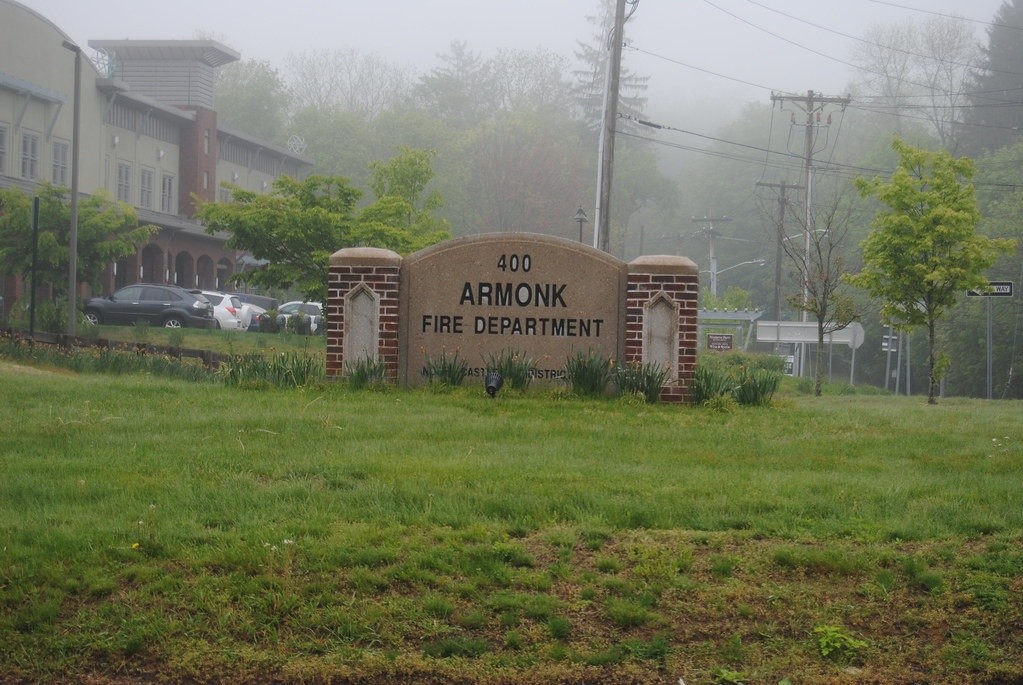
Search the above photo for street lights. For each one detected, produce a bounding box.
[574,204,588,246]
[62,40,81,337]
[772,228,831,321]
[697,258,767,312]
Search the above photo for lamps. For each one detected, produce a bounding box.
[113,136,120,145]
[159,149,164,158]
[261,182,267,189]
[233,173,238,181]
[484,372,504,396]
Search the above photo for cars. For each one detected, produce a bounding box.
[228,290,279,333]
[81,282,219,333]
[278,301,326,335]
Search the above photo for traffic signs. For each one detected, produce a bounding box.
[965,280,1012,297]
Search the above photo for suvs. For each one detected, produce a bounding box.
[198,290,252,337]
